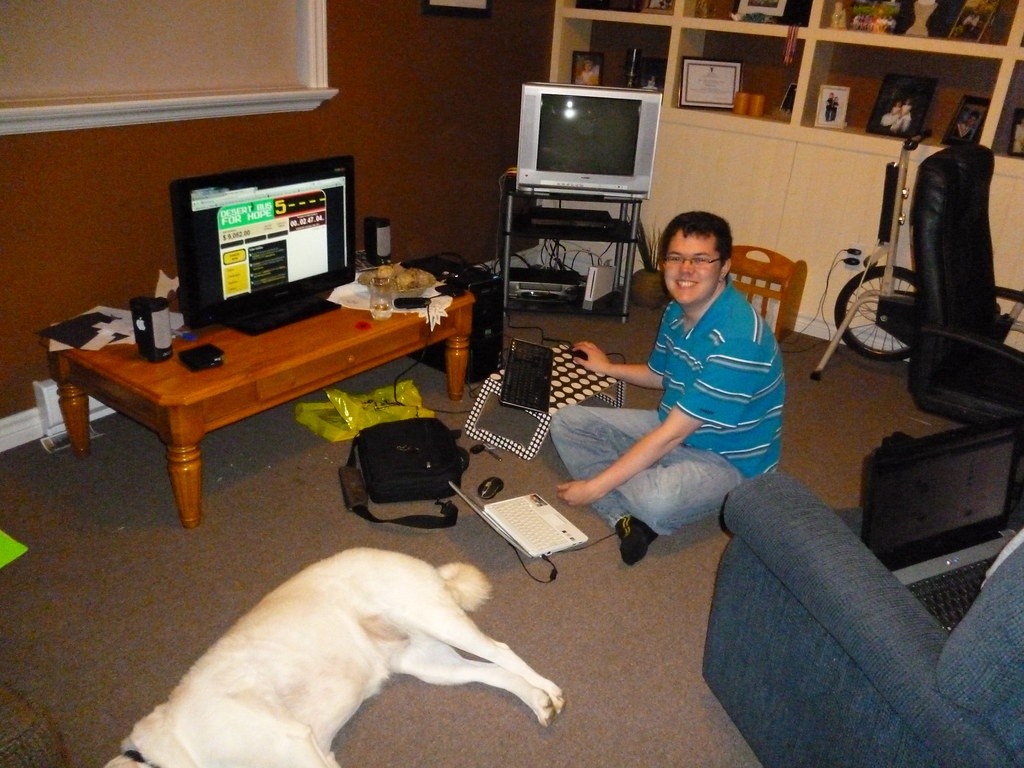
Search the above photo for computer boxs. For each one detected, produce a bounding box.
[400,256,509,384]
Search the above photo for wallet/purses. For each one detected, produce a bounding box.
[180,344,224,372]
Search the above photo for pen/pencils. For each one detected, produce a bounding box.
[172,329,196,341]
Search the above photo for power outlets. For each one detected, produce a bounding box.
[844,243,865,271]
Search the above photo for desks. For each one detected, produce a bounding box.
[40,288,475,528]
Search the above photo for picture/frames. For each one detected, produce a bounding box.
[1007,107,1024,157]
[866,72,939,140]
[738,0,787,16]
[422,0,494,20]
[940,95,991,146]
[814,84,851,129]
[570,51,603,86]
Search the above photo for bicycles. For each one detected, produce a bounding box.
[832,128,1024,362]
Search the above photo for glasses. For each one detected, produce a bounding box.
[666,256,721,269]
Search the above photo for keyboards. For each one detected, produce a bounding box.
[498,338,554,413]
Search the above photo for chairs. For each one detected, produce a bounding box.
[907,145,1024,424]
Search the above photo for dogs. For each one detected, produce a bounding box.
[107,546,565,768]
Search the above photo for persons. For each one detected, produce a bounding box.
[962,14,979,38]
[825,93,839,121]
[551,211,785,564]
[953,112,979,139]
[880,98,913,133]
[853,12,896,33]
[580,60,597,85]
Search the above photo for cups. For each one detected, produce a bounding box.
[369,277,393,319]
[748,93,765,116]
[733,91,749,113]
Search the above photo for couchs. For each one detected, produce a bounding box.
[702,472,1024,768]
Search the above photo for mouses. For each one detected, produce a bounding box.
[568,346,588,361]
[479,477,504,498]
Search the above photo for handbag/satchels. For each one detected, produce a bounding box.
[339,417,469,529]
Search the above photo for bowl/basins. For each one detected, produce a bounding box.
[358,269,435,300]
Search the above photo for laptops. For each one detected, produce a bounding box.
[448,481,589,559]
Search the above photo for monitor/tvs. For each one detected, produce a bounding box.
[169,154,356,336]
[515,81,663,199]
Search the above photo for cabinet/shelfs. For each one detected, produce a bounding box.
[502,175,642,324]
[541,0,1024,363]
